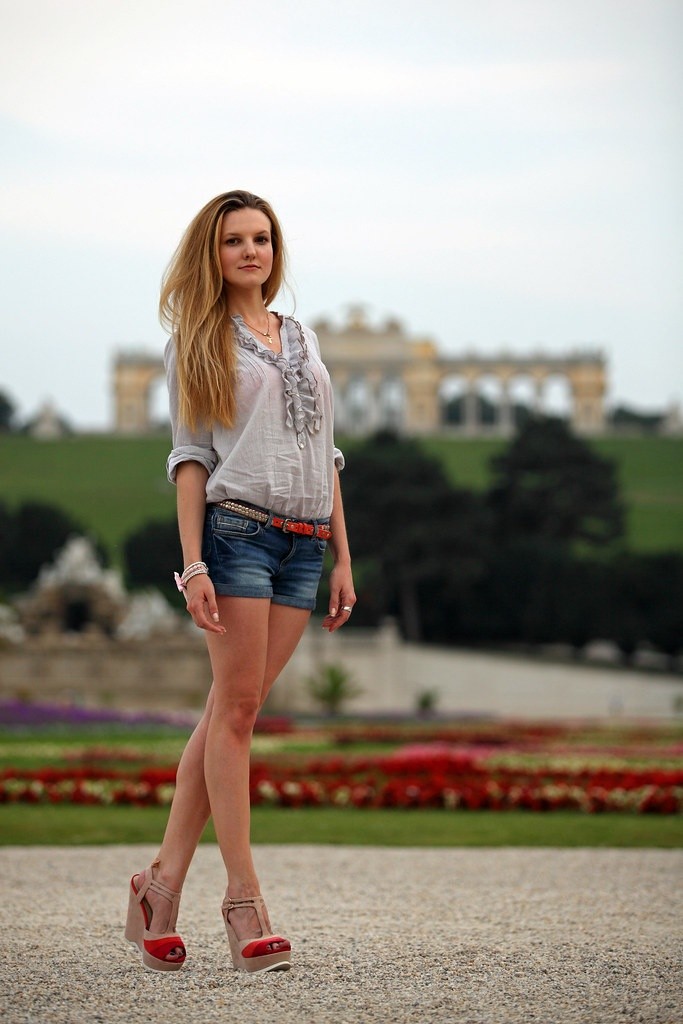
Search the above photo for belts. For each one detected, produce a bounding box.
[215,500,333,540]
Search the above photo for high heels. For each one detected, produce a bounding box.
[124,858,188,971]
[220,885,292,974]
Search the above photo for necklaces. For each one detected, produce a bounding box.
[245,315,272,344]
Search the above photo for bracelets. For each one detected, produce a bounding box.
[174,561,208,601]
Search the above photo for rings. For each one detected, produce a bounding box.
[341,606,352,613]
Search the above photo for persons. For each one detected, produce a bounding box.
[126,189,358,971]
